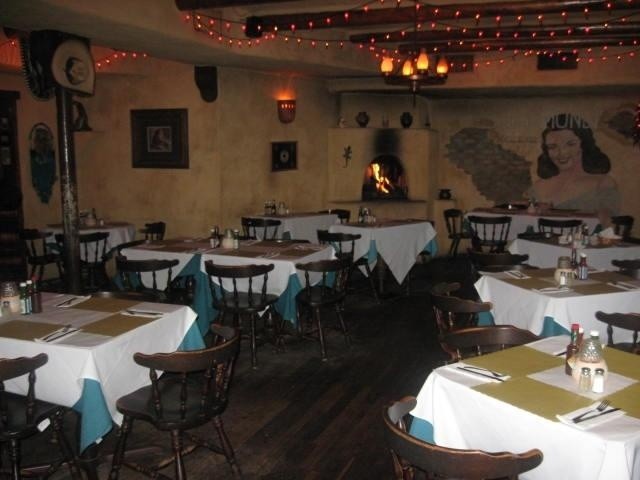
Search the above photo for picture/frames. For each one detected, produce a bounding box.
[130,108,189,169]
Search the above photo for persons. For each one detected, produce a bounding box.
[520,113,621,217]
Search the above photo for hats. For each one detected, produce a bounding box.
[51,39,95,94]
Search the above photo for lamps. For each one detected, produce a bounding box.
[379,1,450,109]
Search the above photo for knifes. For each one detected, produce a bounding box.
[574,408,621,423]
[457,367,505,382]
[46,327,83,342]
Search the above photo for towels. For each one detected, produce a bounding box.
[256,253,280,260]
[531,286,574,294]
[523,334,605,359]
[239,239,261,247]
[52,293,91,308]
[33,326,83,345]
[607,281,639,291]
[433,361,511,384]
[192,248,216,255]
[136,242,167,249]
[120,308,169,318]
[598,225,623,239]
[504,270,532,280]
[555,400,627,430]
[294,244,328,251]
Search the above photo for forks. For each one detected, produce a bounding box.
[572,399,611,421]
[43,324,71,340]
[540,285,570,294]
[125,308,164,316]
[464,366,507,377]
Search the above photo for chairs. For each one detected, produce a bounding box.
[610,258,639,281]
[604,212,634,238]
[107,323,243,480]
[430,279,494,333]
[465,246,538,292]
[0,352,82,480]
[595,309,640,356]
[438,325,544,364]
[115,249,179,294]
[241,216,280,241]
[137,221,166,244]
[204,259,289,370]
[538,218,584,237]
[317,228,382,306]
[55,231,111,284]
[101,241,141,291]
[294,251,355,362]
[467,214,513,253]
[442,208,476,256]
[19,228,66,286]
[381,395,543,479]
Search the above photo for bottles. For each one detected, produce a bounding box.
[578,255,588,280]
[209,229,215,249]
[567,272,573,288]
[579,367,592,392]
[565,324,579,375]
[570,249,578,279]
[579,328,585,347]
[580,330,604,367]
[264,199,293,217]
[559,271,566,288]
[233,229,240,250]
[583,226,589,245]
[26,280,32,314]
[100,218,105,227]
[592,368,604,393]
[18,282,26,315]
[32,276,41,313]
[1,301,11,321]
[215,226,220,247]
